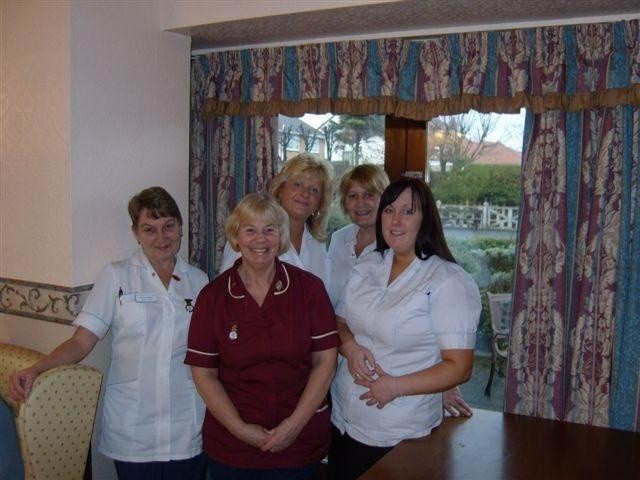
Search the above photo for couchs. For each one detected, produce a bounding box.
[0,343,104,480]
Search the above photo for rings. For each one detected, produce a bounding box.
[355,372,360,377]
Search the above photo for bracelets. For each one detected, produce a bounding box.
[395,377,405,398]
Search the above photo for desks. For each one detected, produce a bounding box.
[356,404,628,478]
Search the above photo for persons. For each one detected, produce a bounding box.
[218,153,336,293]
[328,175,482,480]
[183,191,342,477]
[12,184,211,480]
[327,163,474,420]
